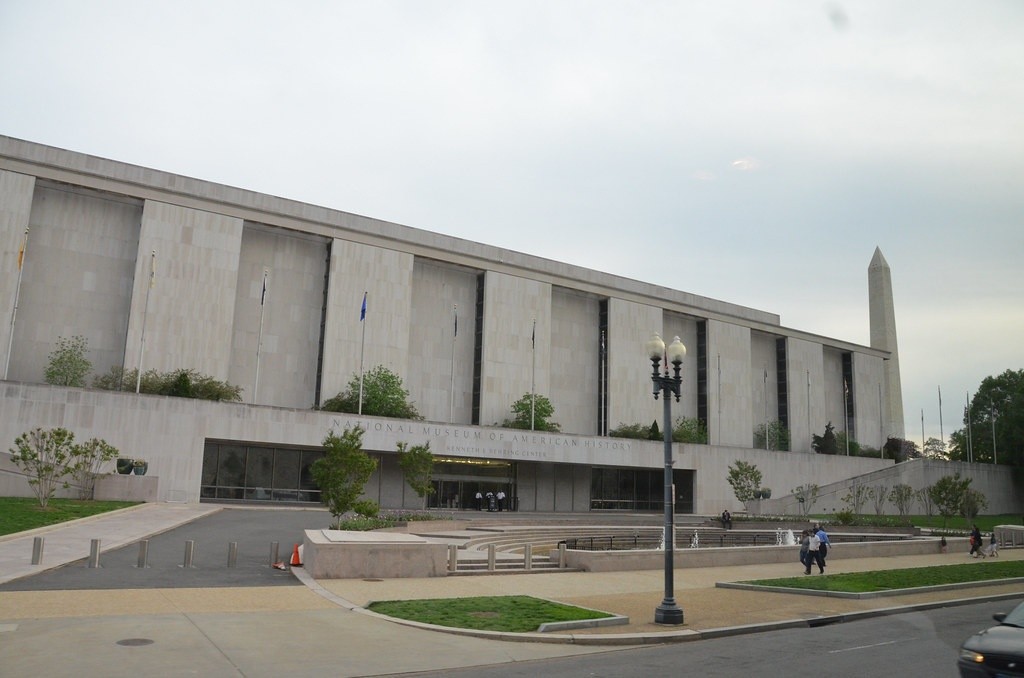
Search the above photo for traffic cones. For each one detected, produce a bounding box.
[272,560,288,571]
[287,542,304,567]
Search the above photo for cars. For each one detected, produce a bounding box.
[955,598,1024,678]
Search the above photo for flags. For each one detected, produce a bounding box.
[763,368,767,383]
[360,293,366,321]
[845,380,848,398]
[261,277,266,306]
[151,257,155,289]
[454,309,457,337]
[18,234,25,270]
[532,324,534,348]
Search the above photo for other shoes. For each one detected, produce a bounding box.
[804,571,810,575]
[819,570,824,574]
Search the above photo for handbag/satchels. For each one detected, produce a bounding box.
[970,535,974,545]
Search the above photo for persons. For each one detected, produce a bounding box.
[486,489,493,511]
[991,533,998,557]
[496,490,505,512]
[722,510,732,529]
[815,525,831,566]
[941,537,948,552]
[475,493,483,511]
[799,530,809,572]
[811,523,820,564]
[968,524,986,559]
[803,529,824,575]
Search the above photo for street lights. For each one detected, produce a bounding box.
[643,330,687,625]
